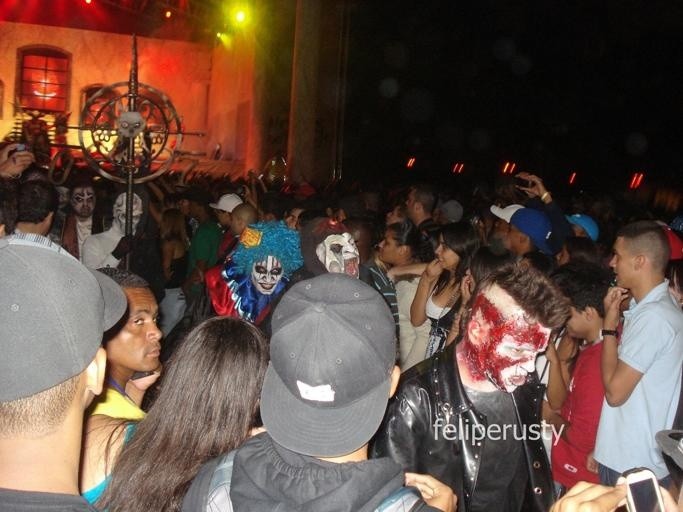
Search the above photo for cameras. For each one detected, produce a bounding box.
[17,142,26,153]
[511,175,537,190]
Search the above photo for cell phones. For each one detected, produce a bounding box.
[616,465,665,511]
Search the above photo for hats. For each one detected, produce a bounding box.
[509,207,563,256]
[491,202,523,223]
[0,230,127,404]
[566,209,600,244]
[259,271,398,458]
[209,194,243,213]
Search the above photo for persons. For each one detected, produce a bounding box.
[0,141,683,511]
